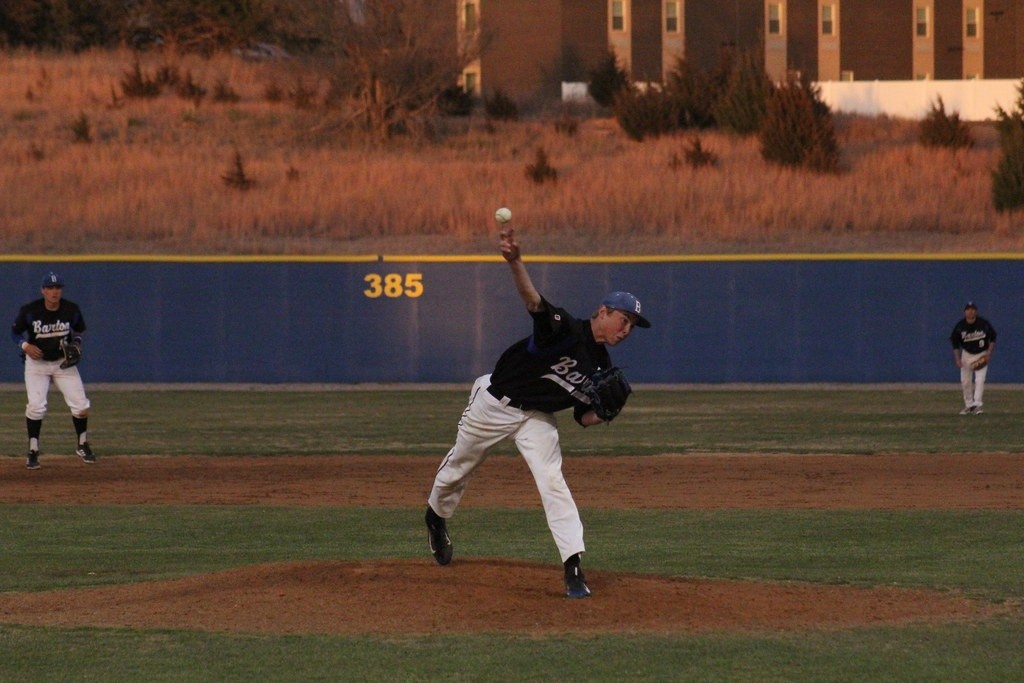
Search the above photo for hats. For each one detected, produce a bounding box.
[964,302,976,312]
[41,271,64,289]
[603,290,651,328]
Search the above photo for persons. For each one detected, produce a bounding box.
[951,301,996,414]
[11,269,96,469]
[424,229,653,598]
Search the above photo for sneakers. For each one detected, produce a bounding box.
[564,567,591,599]
[959,405,975,414]
[975,406,984,415]
[26,449,41,470]
[424,505,453,566]
[75,442,97,464]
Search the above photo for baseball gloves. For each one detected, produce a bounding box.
[585,365,631,422]
[59,346,80,369]
[970,354,991,371]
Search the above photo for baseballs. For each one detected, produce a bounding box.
[495,207,512,223]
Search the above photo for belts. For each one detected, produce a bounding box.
[485,385,531,411]
